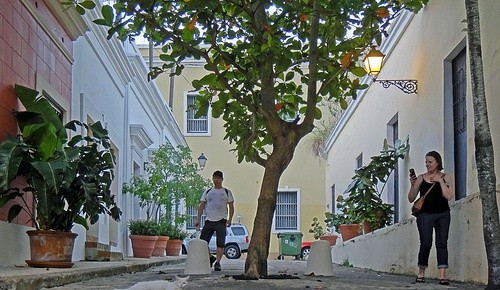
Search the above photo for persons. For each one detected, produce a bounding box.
[194,170,235,271]
[408,150,454,285]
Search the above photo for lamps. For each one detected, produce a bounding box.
[195,153,207,172]
[362,45,418,95]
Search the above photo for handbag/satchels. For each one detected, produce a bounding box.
[411,197,425,216]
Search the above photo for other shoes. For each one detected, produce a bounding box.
[438,279,449,285]
[210,255,217,267]
[214,262,221,271]
[415,276,425,283]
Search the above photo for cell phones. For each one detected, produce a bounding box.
[409,169,417,180]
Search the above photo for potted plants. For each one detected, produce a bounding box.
[309,134,410,245]
[122,142,213,258]
[0,83,123,268]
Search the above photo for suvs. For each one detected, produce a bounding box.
[181,222,251,259]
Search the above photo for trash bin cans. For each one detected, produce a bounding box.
[276,231,303,260]
